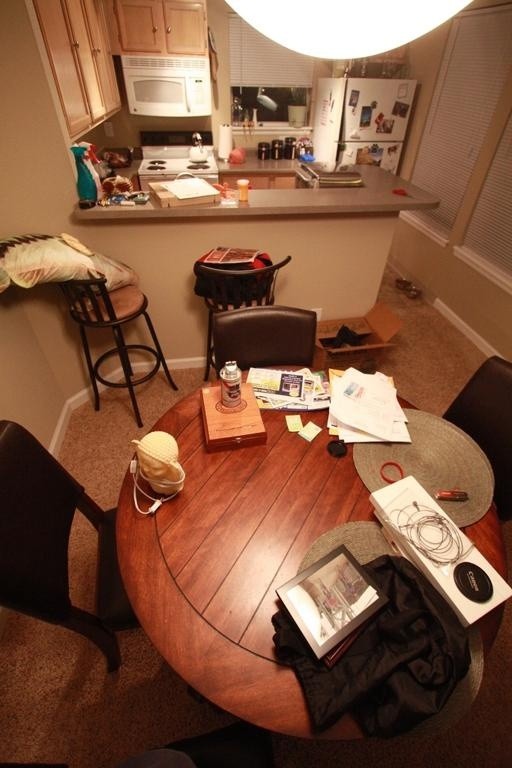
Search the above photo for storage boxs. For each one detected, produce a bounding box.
[312,299,405,373]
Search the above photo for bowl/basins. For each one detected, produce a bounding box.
[395,277,413,291]
[403,285,422,299]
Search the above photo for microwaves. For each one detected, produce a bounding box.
[118,55,214,118]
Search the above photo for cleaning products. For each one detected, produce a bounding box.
[70,140,104,202]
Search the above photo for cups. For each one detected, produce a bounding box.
[237,179,250,202]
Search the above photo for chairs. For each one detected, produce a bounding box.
[438,354,512,527]
[0,420,157,672]
[45,257,320,427]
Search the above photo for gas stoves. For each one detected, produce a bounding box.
[137,155,218,174]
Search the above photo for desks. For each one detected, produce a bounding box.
[111,374,509,742]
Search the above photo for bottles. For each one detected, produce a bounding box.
[270,139,284,160]
[258,141,270,160]
[283,136,296,160]
[231,97,244,127]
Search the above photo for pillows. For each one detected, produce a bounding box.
[0,231,139,302]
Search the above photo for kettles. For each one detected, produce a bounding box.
[188,132,210,163]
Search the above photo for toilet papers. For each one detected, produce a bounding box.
[218,125,233,159]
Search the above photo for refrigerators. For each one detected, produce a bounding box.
[312,76,419,176]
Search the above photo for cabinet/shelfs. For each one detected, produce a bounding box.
[34,1,123,143]
[117,0,208,58]
